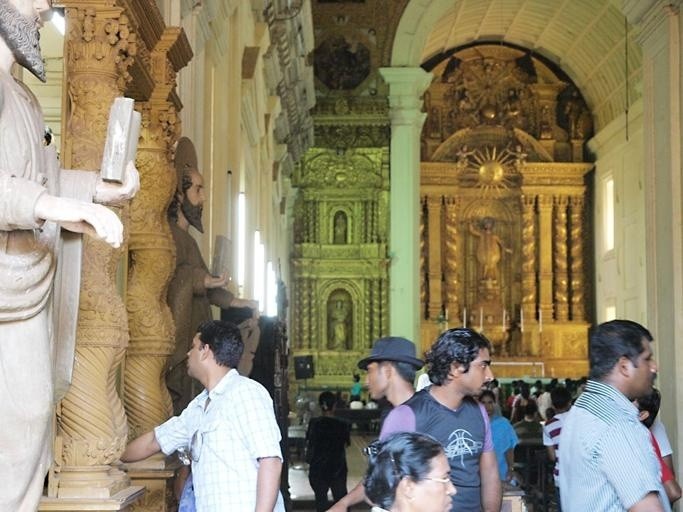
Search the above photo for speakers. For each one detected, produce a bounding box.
[293,355,314,379]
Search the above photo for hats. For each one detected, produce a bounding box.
[357,335,425,370]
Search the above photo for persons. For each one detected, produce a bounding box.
[462,213,515,280]
[555,320,672,512]
[118,320,290,512]
[376,326,505,512]
[423,57,583,165]
[301,392,354,512]
[331,300,347,350]
[333,212,347,239]
[0,0,141,512]
[477,373,680,512]
[360,429,457,512]
[166,132,261,412]
[326,335,420,512]
